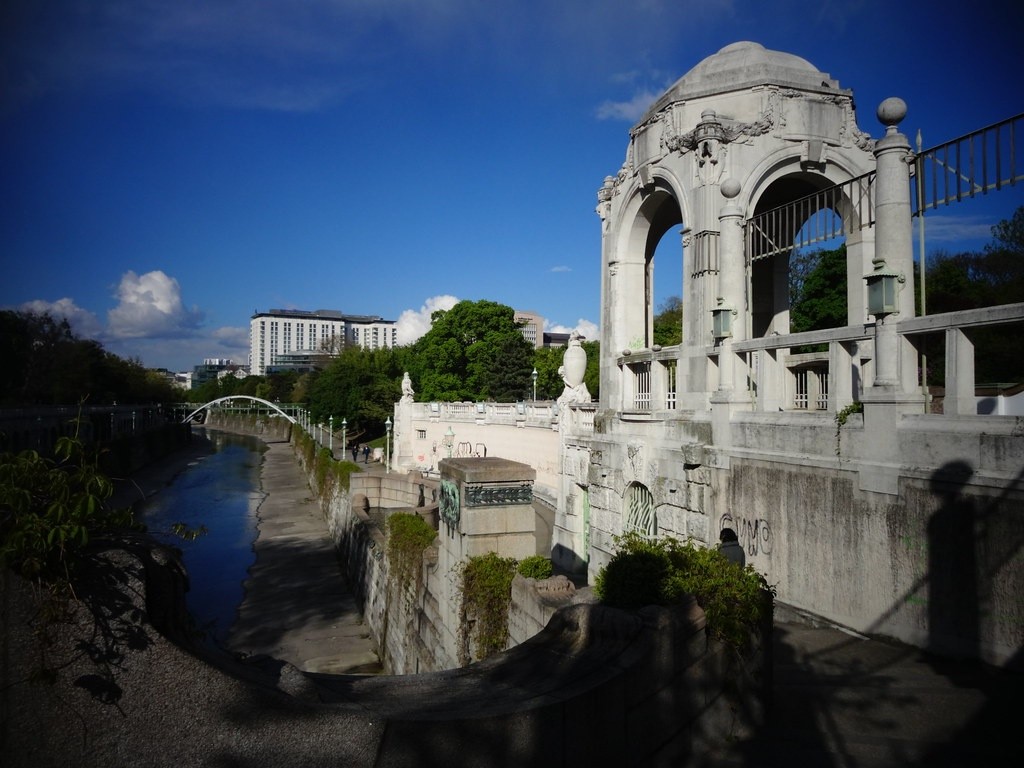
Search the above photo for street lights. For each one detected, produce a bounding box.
[328,415,333,451]
[301,409,303,427]
[304,409,307,431]
[342,417,347,460]
[532,366,539,403]
[384,416,392,475]
[308,411,311,434]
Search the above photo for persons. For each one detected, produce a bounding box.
[352,445,359,462]
[362,443,371,464]
[717,528,746,604]
